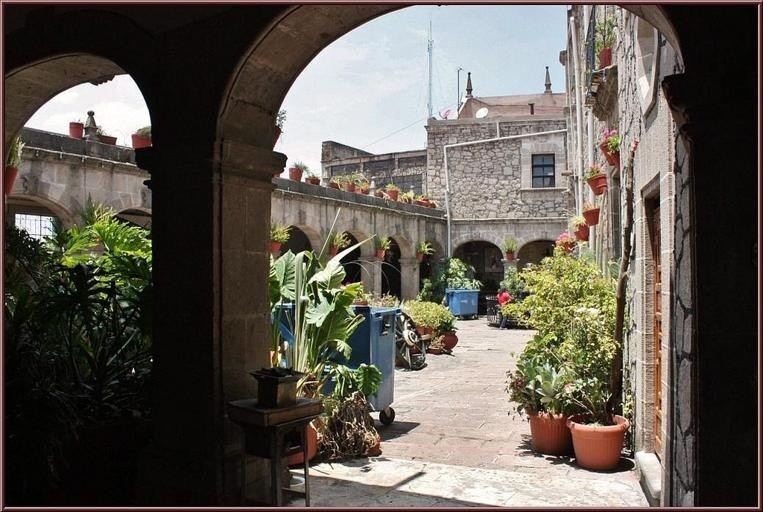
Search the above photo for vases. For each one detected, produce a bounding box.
[66,116,83,137]
[249,365,305,406]
[97,130,117,147]
[556,138,622,255]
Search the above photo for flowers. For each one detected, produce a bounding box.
[591,125,618,145]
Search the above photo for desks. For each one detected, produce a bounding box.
[224,396,327,506]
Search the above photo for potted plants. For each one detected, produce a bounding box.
[402,293,461,358]
[3,133,27,199]
[285,157,438,210]
[270,220,434,267]
[502,352,572,456]
[562,375,630,471]
[501,232,518,260]
[589,10,613,68]
[130,123,152,149]
[269,204,405,465]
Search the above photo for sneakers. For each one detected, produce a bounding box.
[499,326,508,330]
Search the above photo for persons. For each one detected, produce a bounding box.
[493,285,510,330]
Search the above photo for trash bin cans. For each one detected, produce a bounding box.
[445,287,480,320]
[271,303,401,425]
